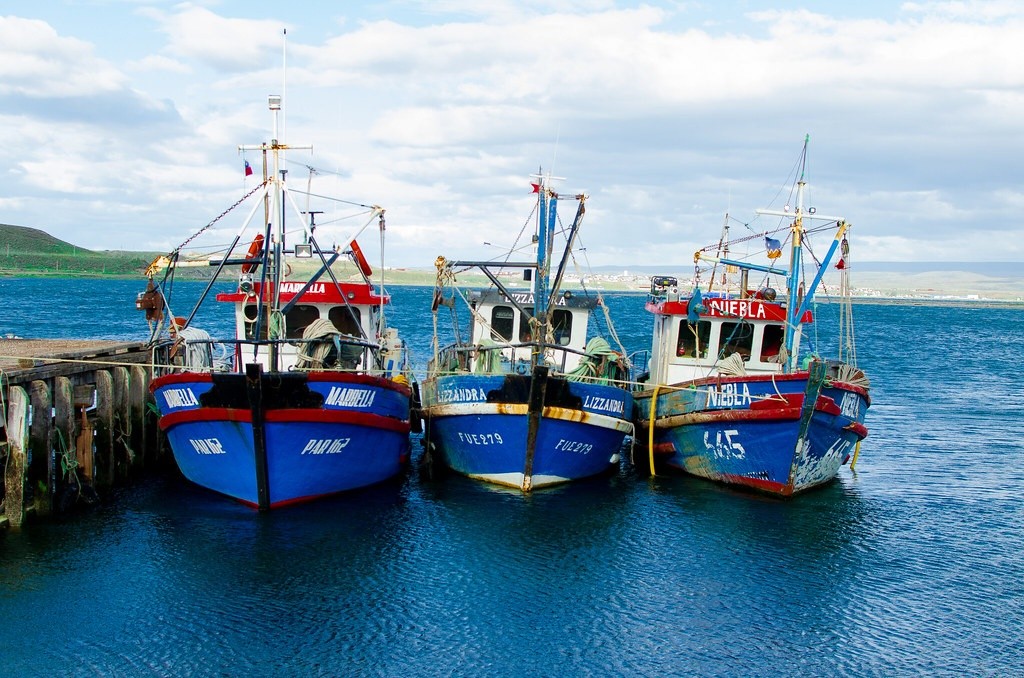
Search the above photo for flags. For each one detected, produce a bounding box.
[245,159,253,176]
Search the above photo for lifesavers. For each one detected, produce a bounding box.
[350,239,372,275]
[240,235,263,275]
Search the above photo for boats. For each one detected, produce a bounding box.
[135,27,420,511]
[630,133,872,499]
[420,166,635,493]
